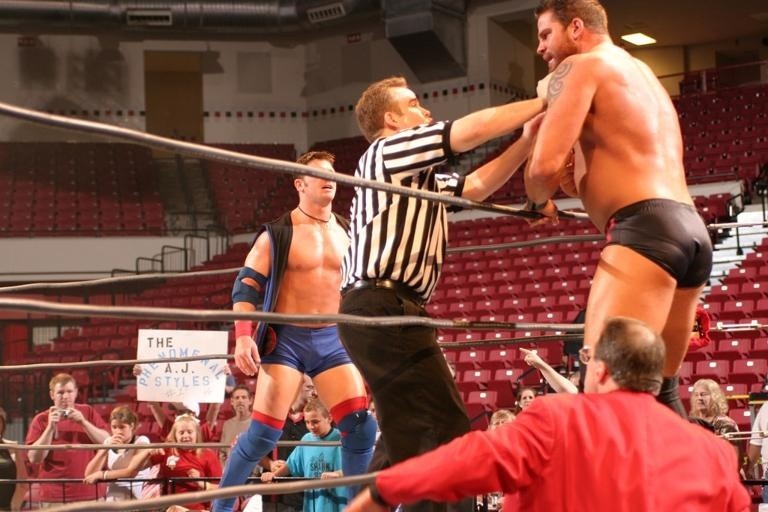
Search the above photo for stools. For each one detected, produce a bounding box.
[0,180,13,185]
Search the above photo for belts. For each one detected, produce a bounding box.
[341,280,428,307]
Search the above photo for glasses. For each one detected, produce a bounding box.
[578,346,594,364]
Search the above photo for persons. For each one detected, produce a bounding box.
[485,408,512,432]
[261,396,350,512]
[337,71,559,512]
[220,385,254,466]
[206,152,379,511]
[335,316,752,512]
[0,410,17,511]
[687,378,743,471]
[81,404,153,506]
[274,373,309,461]
[225,375,235,400]
[513,383,535,414]
[523,0,713,428]
[141,416,222,512]
[130,362,233,446]
[743,394,768,504]
[28,377,111,507]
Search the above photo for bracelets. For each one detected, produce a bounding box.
[103,469,109,480]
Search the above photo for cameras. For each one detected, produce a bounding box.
[55,407,71,417]
